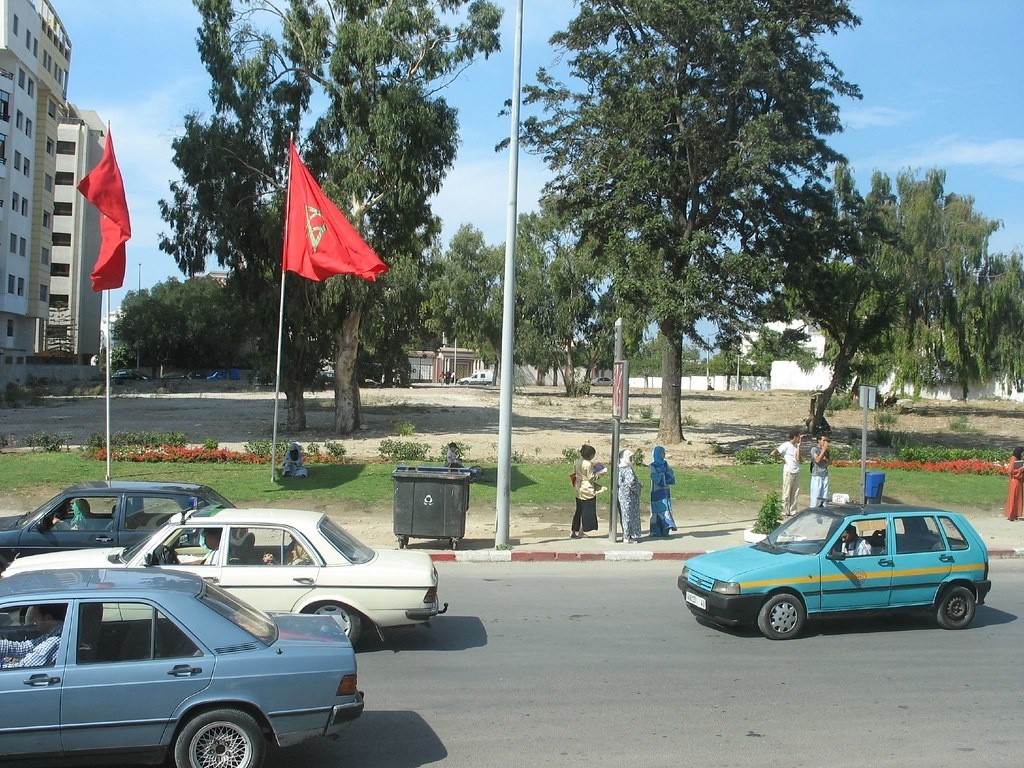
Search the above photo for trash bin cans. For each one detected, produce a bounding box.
[864,472,886,506]
[393,467,468,551]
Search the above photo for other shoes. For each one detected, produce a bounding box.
[578,533,590,538]
[571,534,577,538]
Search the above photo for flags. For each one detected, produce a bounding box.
[73,133,134,290]
[285,146,390,282]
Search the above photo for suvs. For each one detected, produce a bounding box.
[457,368,494,385]
[206,369,243,380]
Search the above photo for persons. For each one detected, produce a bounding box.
[771,431,802,516]
[840,524,872,556]
[810,433,831,507]
[570,444,608,538]
[439,371,454,385]
[649,446,677,537]
[180,529,222,564]
[0,603,68,668]
[446,442,464,468]
[53,499,94,530]
[617,449,644,544]
[261,533,312,565]
[1004,446,1024,520]
[281,442,308,477]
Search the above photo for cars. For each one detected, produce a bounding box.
[0,480,239,576]
[1,567,365,767]
[677,492,991,640]
[591,376,614,386]
[0,508,449,648]
[111,369,154,381]
